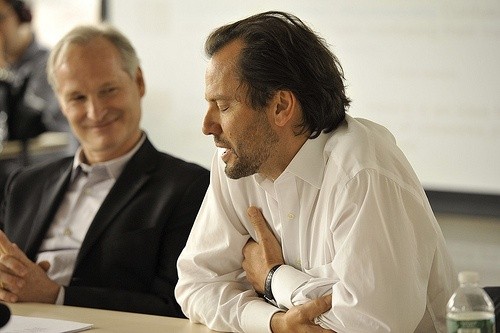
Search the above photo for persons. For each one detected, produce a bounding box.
[1,0,79,168]
[0,27,213,319]
[173,11,459,333]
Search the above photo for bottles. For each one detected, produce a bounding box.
[446,271,496,333]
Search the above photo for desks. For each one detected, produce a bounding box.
[0,299,224,333]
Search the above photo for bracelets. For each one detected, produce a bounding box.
[264,264,281,306]
